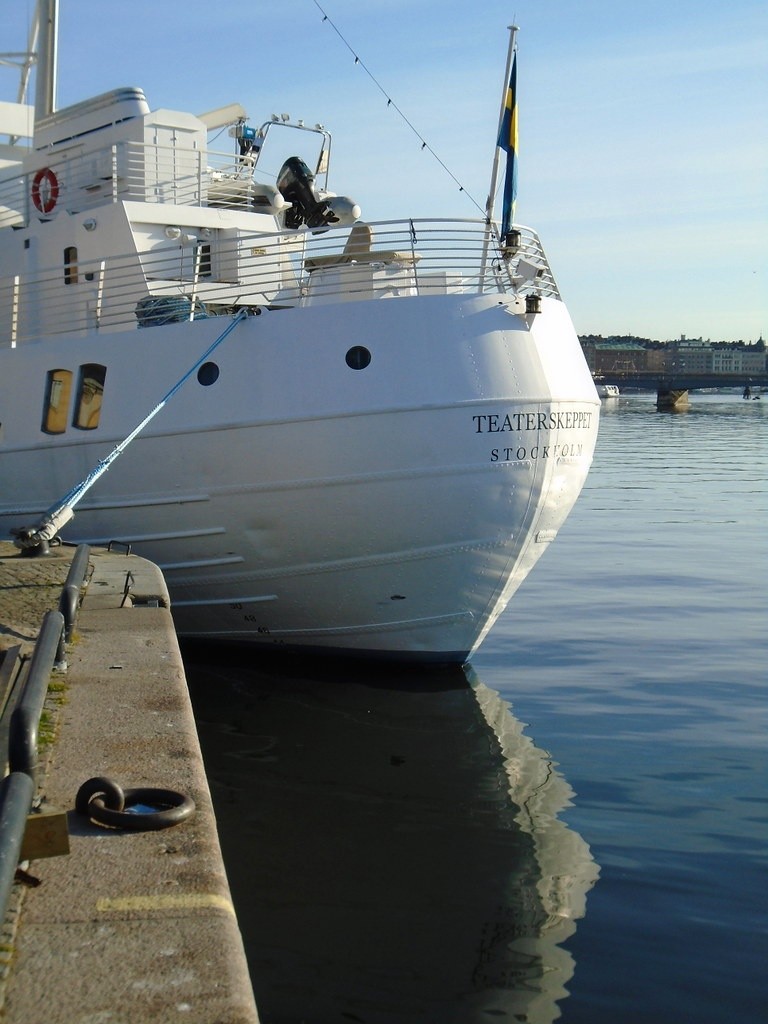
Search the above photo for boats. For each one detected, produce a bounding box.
[0,2,601,674]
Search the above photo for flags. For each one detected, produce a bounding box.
[492,40,520,242]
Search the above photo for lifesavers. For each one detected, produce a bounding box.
[31,169,60,214]
